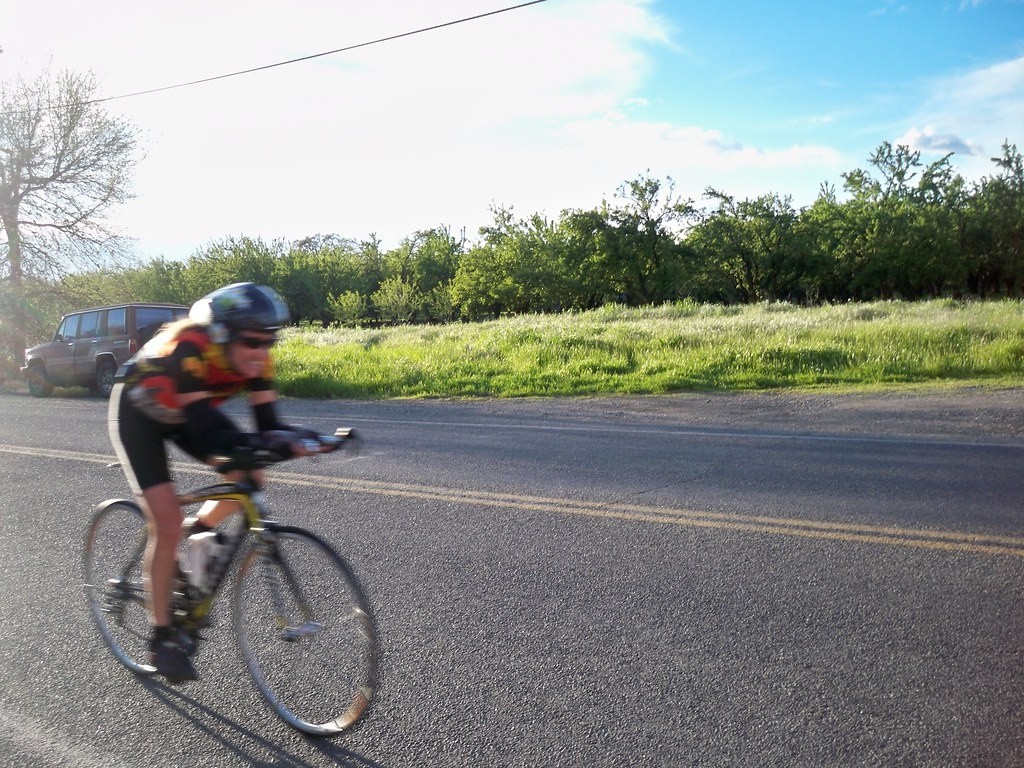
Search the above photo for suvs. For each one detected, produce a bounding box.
[20,303,190,398]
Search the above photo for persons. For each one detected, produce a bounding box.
[108,282,311,683]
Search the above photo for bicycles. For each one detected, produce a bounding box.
[83,428,379,738]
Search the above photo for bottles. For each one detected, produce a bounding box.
[201,533,229,591]
[186,526,216,587]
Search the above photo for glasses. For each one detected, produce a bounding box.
[228,336,279,350]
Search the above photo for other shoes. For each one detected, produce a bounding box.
[147,628,197,683]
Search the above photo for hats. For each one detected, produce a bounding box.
[208,281,289,332]
